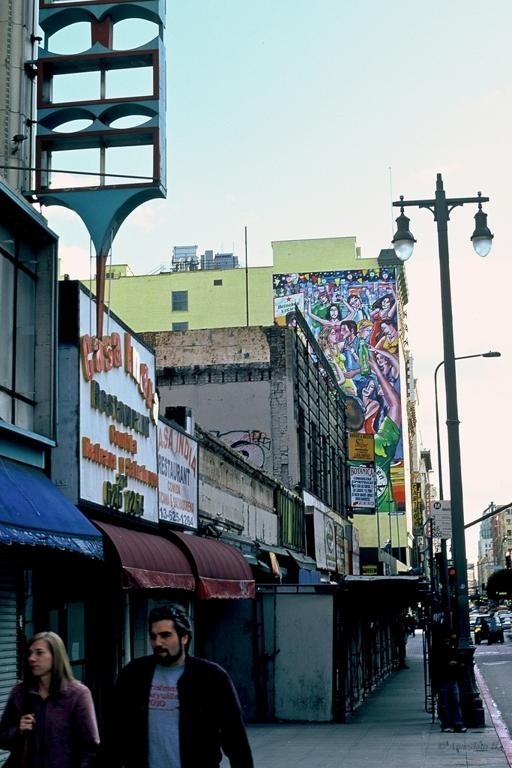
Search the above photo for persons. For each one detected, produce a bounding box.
[487,612,497,646]
[428,631,468,733]
[307,283,402,513]
[395,615,418,669]
[105,603,254,768]
[0,631,101,768]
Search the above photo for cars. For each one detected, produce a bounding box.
[469,605,512,645]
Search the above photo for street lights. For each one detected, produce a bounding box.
[435,351,501,644]
[390,173,503,728]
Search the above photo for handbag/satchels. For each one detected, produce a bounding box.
[0,730,34,768]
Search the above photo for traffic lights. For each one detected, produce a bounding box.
[448,565,457,585]
